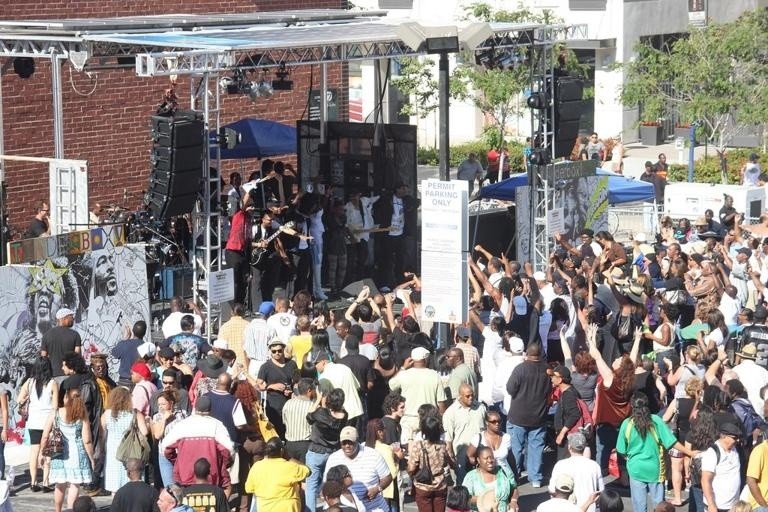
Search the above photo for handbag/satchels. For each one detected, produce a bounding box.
[414,462,431,484]
[18,399,30,421]
[116,429,151,463]
[42,426,63,459]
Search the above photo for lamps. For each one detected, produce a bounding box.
[476,37,523,73]
[220,60,295,101]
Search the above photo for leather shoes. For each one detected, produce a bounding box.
[42,486,54,492]
[30,485,41,491]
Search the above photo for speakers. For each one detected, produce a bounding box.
[534,69,584,159]
[145,109,204,222]
[468,204,516,270]
[340,276,395,300]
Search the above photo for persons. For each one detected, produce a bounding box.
[0,133,768,511]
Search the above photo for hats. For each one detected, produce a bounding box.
[58,301,431,440]
[456,326,470,340]
[511,218,766,492]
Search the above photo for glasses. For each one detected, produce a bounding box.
[487,419,503,425]
[166,485,177,508]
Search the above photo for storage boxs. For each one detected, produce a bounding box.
[156,265,200,300]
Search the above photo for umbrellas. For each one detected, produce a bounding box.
[193,114,301,208]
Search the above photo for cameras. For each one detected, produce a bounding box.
[701,331,705,338]
[284,383,291,390]
[183,303,190,309]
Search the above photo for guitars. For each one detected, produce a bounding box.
[247,221,294,266]
[345,224,400,244]
